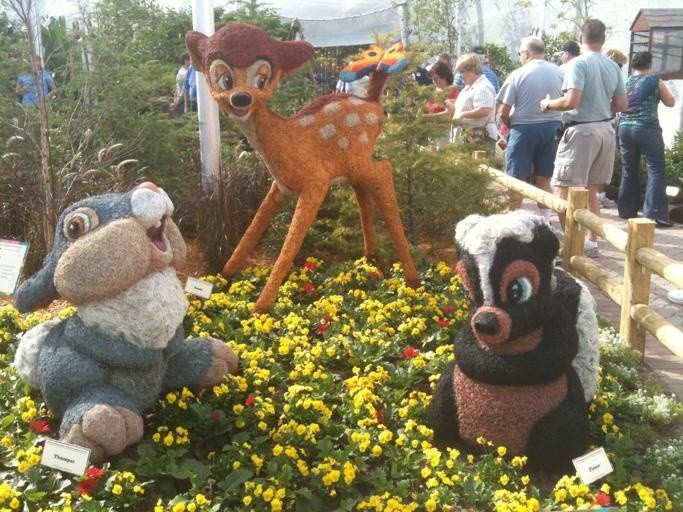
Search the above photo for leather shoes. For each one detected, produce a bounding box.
[655,218,672,228]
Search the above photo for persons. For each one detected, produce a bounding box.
[183,64,197,115]
[412,36,628,225]
[538,18,628,257]
[167,52,190,114]
[616,51,675,228]
[15,54,58,102]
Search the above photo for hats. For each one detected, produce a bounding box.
[554,41,579,56]
[470,45,484,54]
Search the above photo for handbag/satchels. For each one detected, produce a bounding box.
[463,126,489,146]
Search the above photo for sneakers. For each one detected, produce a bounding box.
[583,241,600,257]
[598,196,615,207]
